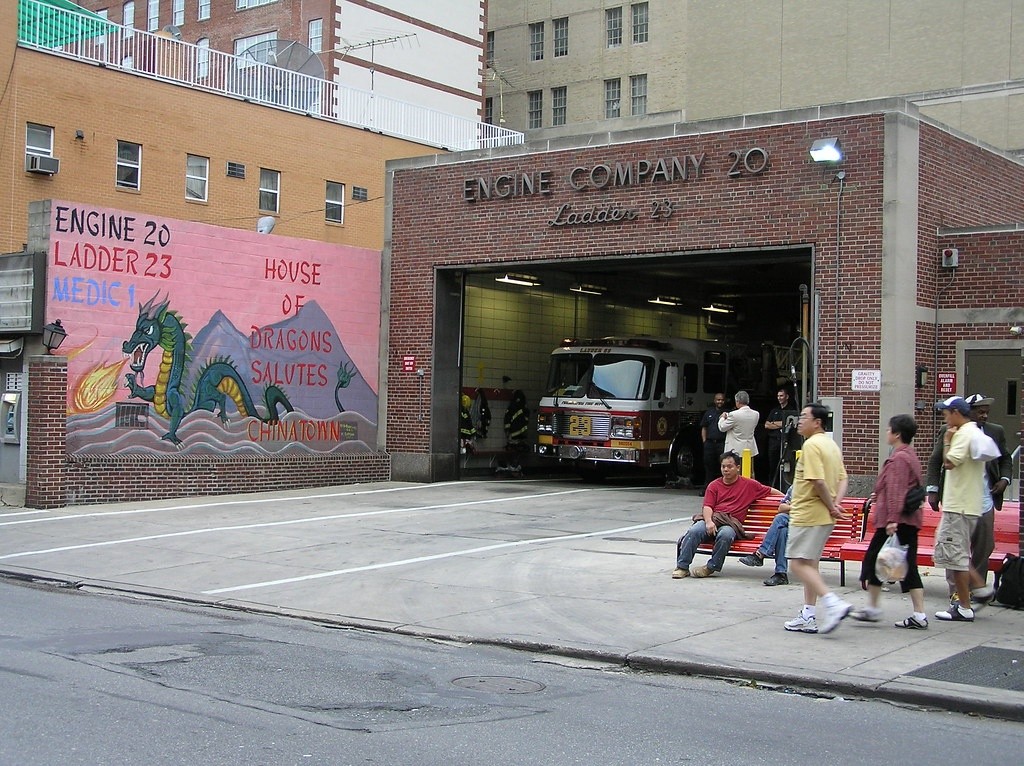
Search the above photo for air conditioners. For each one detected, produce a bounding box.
[26,155,60,175]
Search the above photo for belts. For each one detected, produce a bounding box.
[709,439,721,443]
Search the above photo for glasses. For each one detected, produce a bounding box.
[886,429,892,432]
[798,416,815,420]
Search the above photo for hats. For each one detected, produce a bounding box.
[934,396,970,416]
[965,393,994,405]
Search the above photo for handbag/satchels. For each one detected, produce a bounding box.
[900,457,925,515]
[875,533,908,584]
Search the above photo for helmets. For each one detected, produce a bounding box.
[462,395,470,407]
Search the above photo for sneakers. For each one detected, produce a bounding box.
[739,551,764,566]
[818,598,853,634]
[689,564,714,577]
[764,573,788,585]
[895,616,928,629]
[935,605,975,621]
[847,609,878,622]
[672,567,689,577]
[784,609,818,632]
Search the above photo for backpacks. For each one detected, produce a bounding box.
[988,553,1024,611]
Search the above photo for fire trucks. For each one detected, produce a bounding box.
[533,333,809,486]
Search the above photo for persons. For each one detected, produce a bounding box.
[848,414,929,631]
[926,393,1013,622]
[698,391,760,497]
[765,385,797,494]
[672,449,785,579]
[739,403,853,634]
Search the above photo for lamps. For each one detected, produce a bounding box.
[648,294,684,307]
[701,303,735,314]
[41,319,68,355]
[809,137,842,163]
[494,272,545,288]
[1009,326,1023,335]
[570,281,608,296]
[75,130,85,140]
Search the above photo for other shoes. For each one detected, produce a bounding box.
[699,490,705,496]
[970,586,996,612]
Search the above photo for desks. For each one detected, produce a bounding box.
[460,451,514,468]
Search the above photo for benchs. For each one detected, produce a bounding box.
[691,495,867,587]
[840,498,1020,605]
